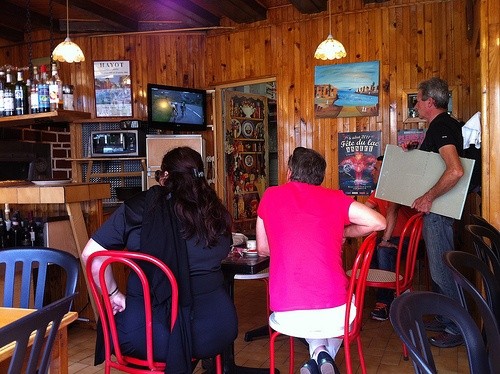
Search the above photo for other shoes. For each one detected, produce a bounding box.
[300,359,318,374]
[202,357,229,374]
[317,351,340,374]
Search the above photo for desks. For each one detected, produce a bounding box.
[202,254,280,374]
[0,307,79,374]
[0,184,112,322]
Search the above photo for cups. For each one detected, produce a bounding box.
[246,240,256,250]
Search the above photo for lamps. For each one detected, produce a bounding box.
[314,0,347,61]
[51,0,85,63]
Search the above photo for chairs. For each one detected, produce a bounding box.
[0,248,79,310]
[466,225,500,348]
[0,291,80,374]
[268,232,379,374]
[86,251,222,374]
[390,291,491,374]
[345,212,425,361]
[442,251,500,374]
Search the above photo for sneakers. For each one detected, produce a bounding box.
[369,302,390,320]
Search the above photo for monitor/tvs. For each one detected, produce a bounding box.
[147,83,206,130]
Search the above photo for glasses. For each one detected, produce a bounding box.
[155,170,165,183]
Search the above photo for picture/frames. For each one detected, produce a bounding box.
[402,86,458,122]
[92,60,134,118]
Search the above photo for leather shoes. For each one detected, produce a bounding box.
[429,329,464,347]
[424,318,454,331]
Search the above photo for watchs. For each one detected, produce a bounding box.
[108,287,120,299]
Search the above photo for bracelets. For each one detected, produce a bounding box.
[382,238,389,242]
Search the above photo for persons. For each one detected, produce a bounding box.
[105,77,112,88]
[256,146,387,374]
[365,157,417,321]
[171,99,186,117]
[80,146,238,357]
[400,79,468,347]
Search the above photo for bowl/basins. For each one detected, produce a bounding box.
[245,252,257,257]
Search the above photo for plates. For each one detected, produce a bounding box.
[32,180,70,186]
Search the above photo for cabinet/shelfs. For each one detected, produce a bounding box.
[225,91,269,223]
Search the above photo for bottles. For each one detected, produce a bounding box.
[14,69,28,116]
[22,210,38,249]
[4,68,15,117]
[29,66,40,114]
[7,211,23,248]
[0,69,6,117]
[4,208,11,231]
[38,65,50,113]
[0,209,7,251]
[49,64,63,112]
